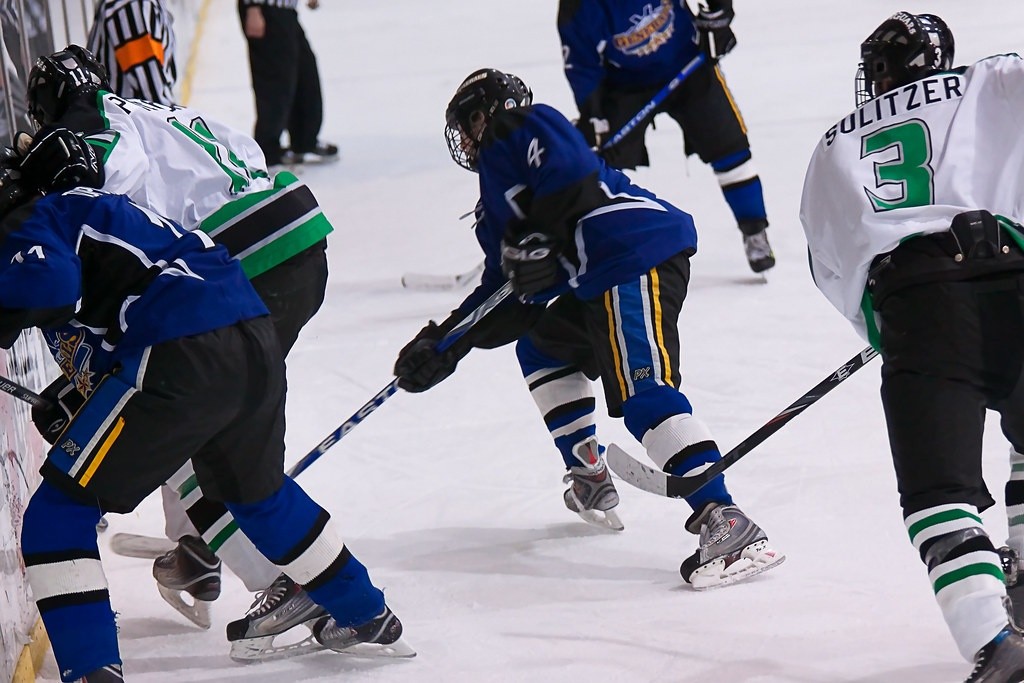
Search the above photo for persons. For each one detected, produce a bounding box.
[557,0,775,274]
[0,126,402,683]
[0,0,176,165]
[24,43,334,597]
[236,0,338,168]
[393,68,769,582]
[800,11,1024,683]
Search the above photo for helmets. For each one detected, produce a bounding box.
[444,69,532,171]
[854,10,956,107]
[27,44,111,132]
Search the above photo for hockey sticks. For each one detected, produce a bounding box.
[602,52,706,150]
[0,374,47,409]
[111,280,516,559]
[606,347,882,500]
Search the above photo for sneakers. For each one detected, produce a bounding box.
[226,575,327,662]
[564,464,623,532]
[262,139,339,169]
[674,507,787,592]
[742,228,777,286]
[312,606,417,661]
[153,535,222,629]
[961,582,1024,683]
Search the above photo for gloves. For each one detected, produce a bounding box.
[690,5,738,61]
[501,225,563,294]
[13,127,104,195]
[394,318,473,393]
[28,374,86,443]
